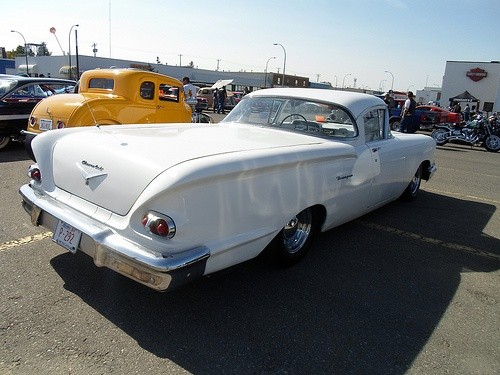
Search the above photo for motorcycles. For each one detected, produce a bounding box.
[430,110,500,152]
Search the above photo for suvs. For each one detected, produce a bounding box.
[376,95,404,132]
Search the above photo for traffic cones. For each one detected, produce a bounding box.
[10,30,28,65]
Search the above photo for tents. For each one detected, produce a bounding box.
[449,91,480,111]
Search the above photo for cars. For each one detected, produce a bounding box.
[197,86,213,106]
[0,73,78,152]
[415,105,463,124]
[170,88,209,113]
[20,65,198,163]
[18,87,436,295]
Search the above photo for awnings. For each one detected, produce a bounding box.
[19,64,37,73]
[59,66,76,74]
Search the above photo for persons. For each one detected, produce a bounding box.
[19,73,47,91]
[182,77,250,115]
[384,92,487,134]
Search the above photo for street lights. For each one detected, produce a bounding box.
[266,56,277,73]
[385,71,395,89]
[68,24,79,55]
[342,73,352,87]
[273,43,287,87]
